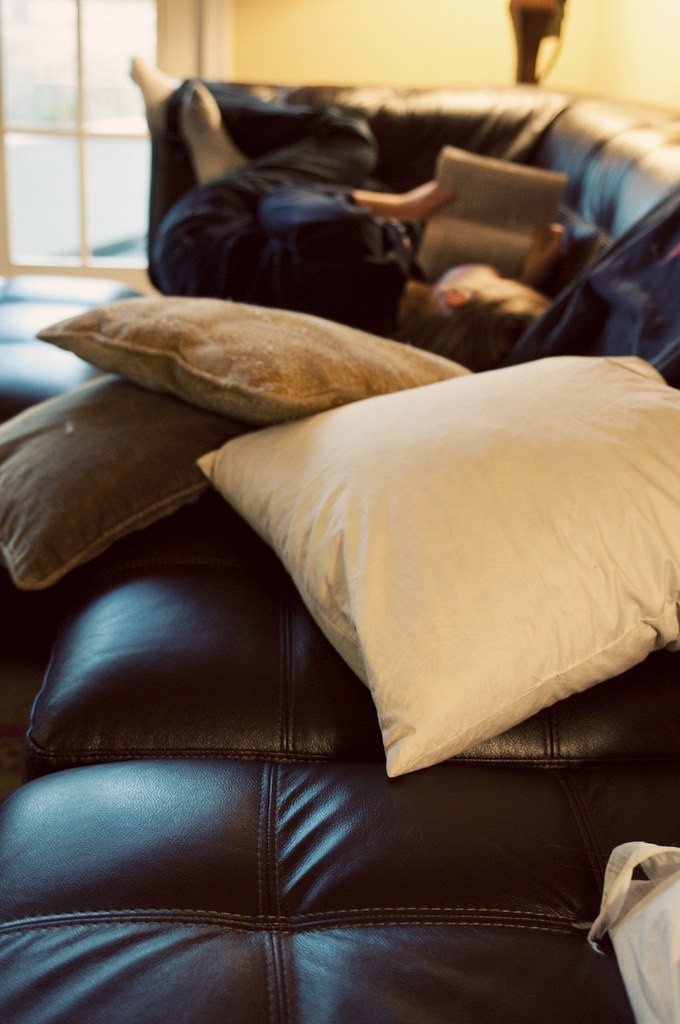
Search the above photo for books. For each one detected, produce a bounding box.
[416,144,569,283]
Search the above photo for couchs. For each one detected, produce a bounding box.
[1,80,680,1024]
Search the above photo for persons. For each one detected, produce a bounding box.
[129,55,564,374]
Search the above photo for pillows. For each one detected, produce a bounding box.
[0,372,263,589]
[35,296,475,422]
[195,356,679,778]
